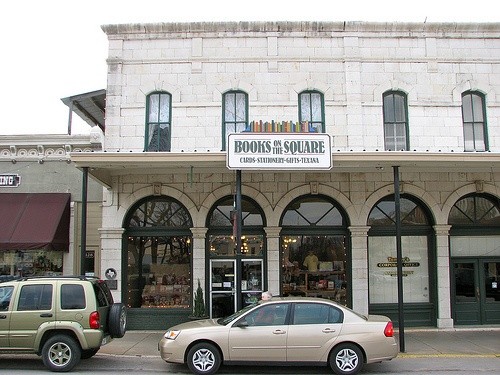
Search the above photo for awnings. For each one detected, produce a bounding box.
[0,193,70,251]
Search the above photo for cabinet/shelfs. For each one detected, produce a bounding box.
[141,285,190,308]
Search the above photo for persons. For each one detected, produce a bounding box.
[303,248,319,271]
[452,274,500,301]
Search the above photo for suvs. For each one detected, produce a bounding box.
[1,273,128,372]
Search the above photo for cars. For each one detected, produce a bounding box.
[158,296,399,374]
